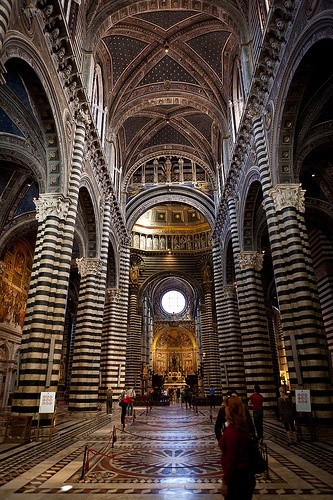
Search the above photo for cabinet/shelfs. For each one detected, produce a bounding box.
[36,413,57,442]
[4,415,32,443]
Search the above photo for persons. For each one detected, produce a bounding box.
[215,390,259,494]
[165,356,184,376]
[249,385,264,444]
[218,396,267,500]
[277,387,319,444]
[105,370,215,423]
